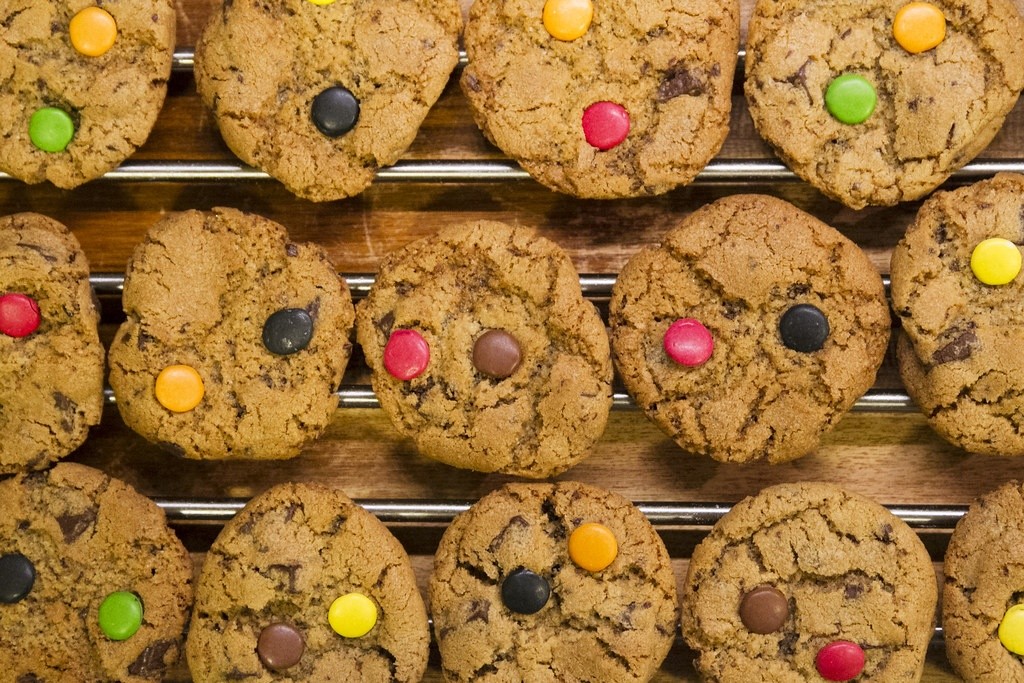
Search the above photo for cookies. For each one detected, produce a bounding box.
[0,0,1024,683]
